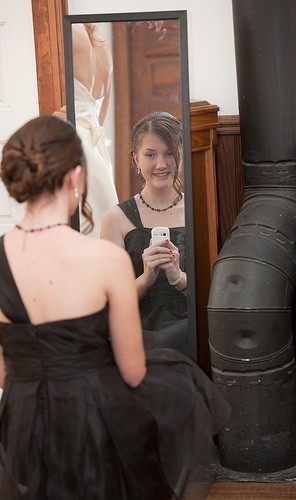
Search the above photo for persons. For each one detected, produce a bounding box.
[98,111,187,356]
[1,117,232,500]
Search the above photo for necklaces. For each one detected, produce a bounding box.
[13,222,70,251]
[139,190,182,212]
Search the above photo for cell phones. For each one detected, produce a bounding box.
[151,227,170,245]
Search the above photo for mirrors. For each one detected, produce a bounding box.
[62,9,197,363]
